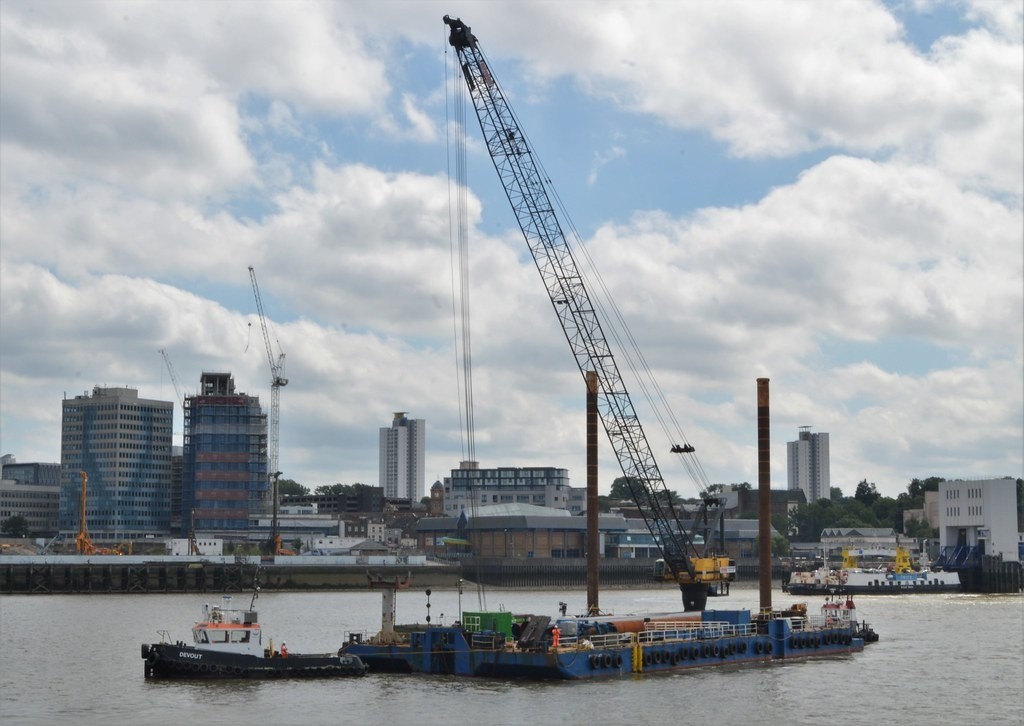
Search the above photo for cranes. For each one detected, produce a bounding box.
[159,349,185,412]
[242,264,287,504]
[442,13,737,610]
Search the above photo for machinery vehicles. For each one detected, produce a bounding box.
[76,471,133,555]
[261,473,296,559]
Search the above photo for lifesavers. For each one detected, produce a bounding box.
[793,632,852,649]
[755,640,764,654]
[602,654,612,668]
[808,584,816,592]
[139,642,369,678]
[590,655,601,669]
[613,653,623,668]
[765,642,773,654]
[643,640,748,666]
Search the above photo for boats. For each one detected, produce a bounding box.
[336,572,875,681]
[788,540,1024,594]
[142,595,366,679]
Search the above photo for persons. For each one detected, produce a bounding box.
[581,639,594,650]
[608,622,618,633]
[552,625,561,647]
[282,640,287,659]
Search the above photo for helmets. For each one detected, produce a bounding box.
[554,625,558,628]
